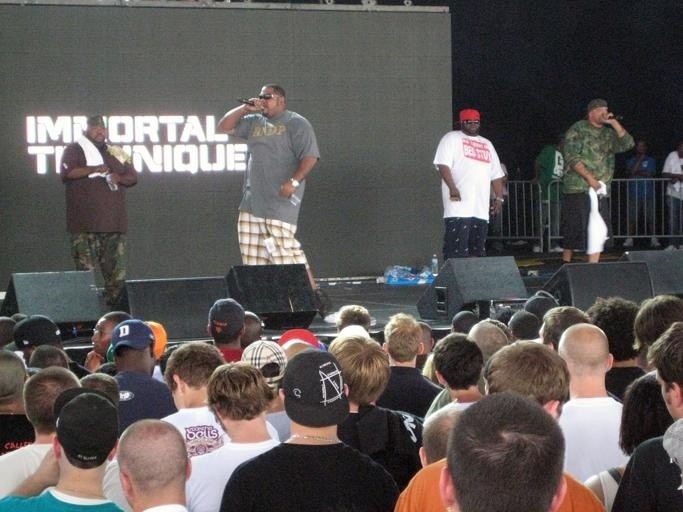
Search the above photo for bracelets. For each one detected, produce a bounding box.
[495,197,504,203]
[290,177,298,188]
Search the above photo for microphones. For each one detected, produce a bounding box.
[235,95,264,110]
[605,114,626,122]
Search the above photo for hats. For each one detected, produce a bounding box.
[456,110,481,121]
[11,314,64,347]
[278,349,351,429]
[55,388,119,469]
[239,338,288,387]
[584,98,608,119]
[109,320,158,355]
[205,298,246,337]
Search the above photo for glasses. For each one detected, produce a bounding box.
[462,119,481,125]
[88,326,114,336]
[257,93,281,99]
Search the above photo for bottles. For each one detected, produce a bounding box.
[431,254,438,276]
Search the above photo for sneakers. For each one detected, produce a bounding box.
[663,244,677,251]
[548,246,565,253]
[313,288,332,317]
[621,236,634,247]
[531,243,542,253]
[677,244,683,249]
[650,238,660,248]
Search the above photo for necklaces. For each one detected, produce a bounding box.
[61,486,106,499]
[291,434,340,443]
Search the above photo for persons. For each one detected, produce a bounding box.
[215,84,333,320]
[529,134,566,254]
[659,140,683,252]
[623,140,662,251]
[432,108,507,260]
[0,296,682,509]
[556,97,636,264]
[60,116,138,314]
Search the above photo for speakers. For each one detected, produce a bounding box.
[415,255,530,322]
[619,249,683,297]
[0,270,102,338]
[458,297,560,323]
[226,264,320,329]
[538,261,654,312]
[110,276,230,342]
